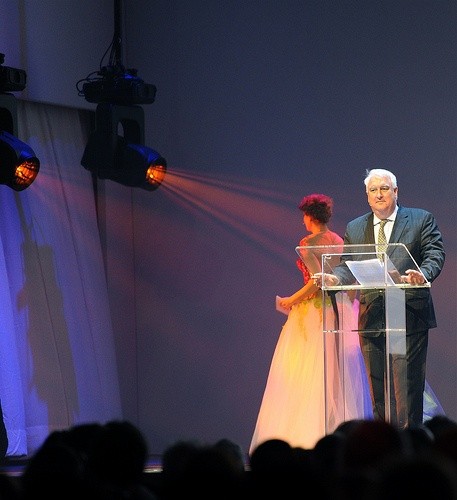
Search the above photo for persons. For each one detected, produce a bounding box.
[312,169,445,431]
[248,194,446,453]
[0,407,457,500]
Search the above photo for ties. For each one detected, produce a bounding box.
[377,220,388,261]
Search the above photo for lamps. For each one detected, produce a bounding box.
[77,1,166,192]
[0,53,40,192]
